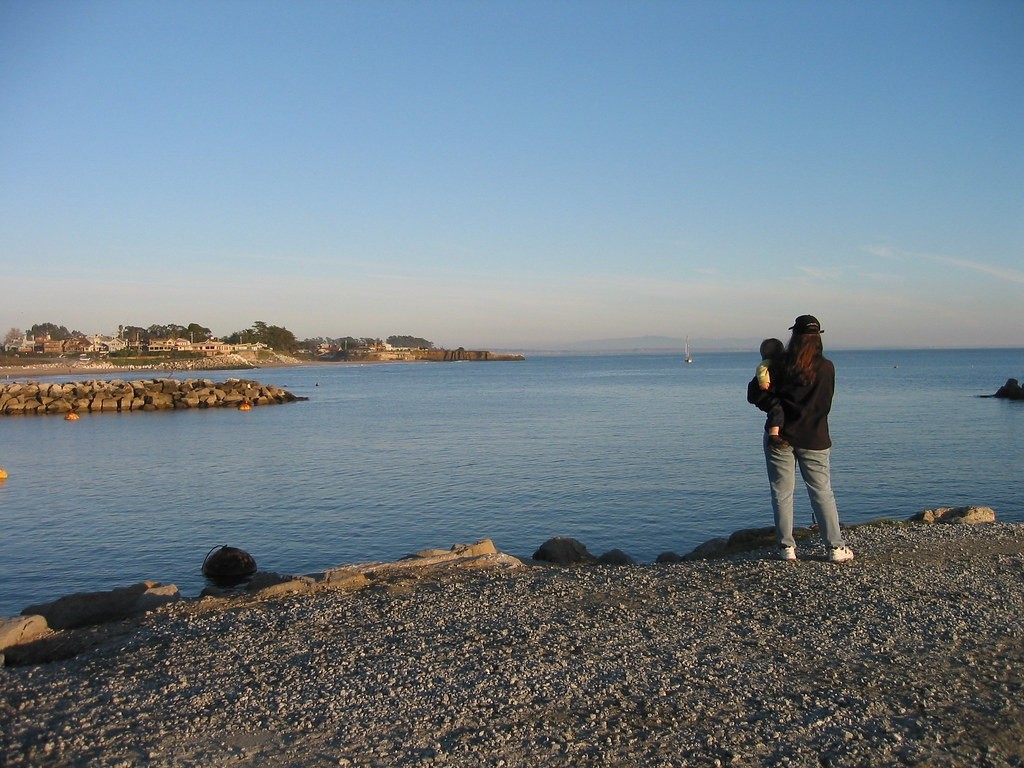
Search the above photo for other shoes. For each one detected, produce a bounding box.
[767,435,794,455]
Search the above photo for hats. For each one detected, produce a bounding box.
[788,315,824,334]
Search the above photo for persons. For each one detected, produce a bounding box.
[747,315,854,562]
[755,338,789,450]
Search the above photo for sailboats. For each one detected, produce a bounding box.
[685,336,693,363]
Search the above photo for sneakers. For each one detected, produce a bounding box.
[829,545,854,561]
[780,546,796,560]
